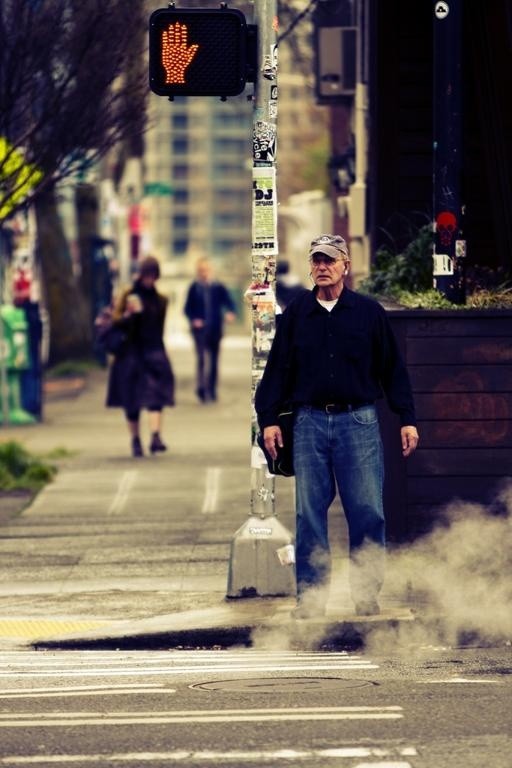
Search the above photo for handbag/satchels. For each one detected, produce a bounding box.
[96,321,126,353]
[258,414,299,479]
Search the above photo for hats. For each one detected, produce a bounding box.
[306,235,346,258]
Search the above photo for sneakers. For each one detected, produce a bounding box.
[290,596,329,620]
[131,435,168,458]
[354,598,381,620]
[193,386,219,401]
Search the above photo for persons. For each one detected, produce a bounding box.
[185,256,240,404]
[104,255,175,458]
[254,234,420,620]
[275,260,307,314]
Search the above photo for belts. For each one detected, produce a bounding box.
[300,400,372,416]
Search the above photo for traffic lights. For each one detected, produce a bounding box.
[149,6,245,96]
